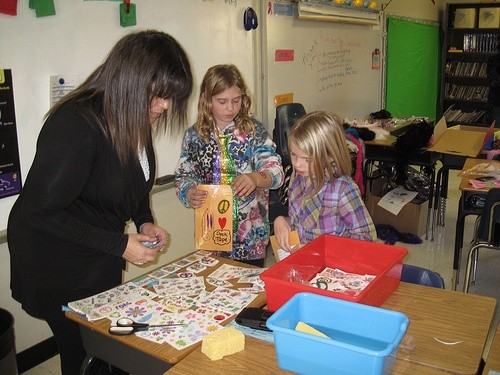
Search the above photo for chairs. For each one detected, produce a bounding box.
[463,187,500,294]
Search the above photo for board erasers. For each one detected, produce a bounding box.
[156,175,175,185]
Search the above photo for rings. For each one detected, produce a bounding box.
[159,246,165,252]
[139,262,144,266]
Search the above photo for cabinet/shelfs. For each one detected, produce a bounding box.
[438,3,500,128]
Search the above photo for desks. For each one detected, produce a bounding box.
[262,281,498,375]
[163,321,455,375]
[430,146,500,242]
[63,250,262,375]
[451,158,500,291]
[362,116,442,240]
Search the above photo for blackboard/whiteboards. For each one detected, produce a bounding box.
[0,0,386,244]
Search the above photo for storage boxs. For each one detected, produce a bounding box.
[426,115,500,158]
[265,292,410,375]
[370,190,433,237]
[259,232,410,312]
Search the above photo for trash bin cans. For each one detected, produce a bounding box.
[0,307,18,375]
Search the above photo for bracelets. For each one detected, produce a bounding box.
[259,171,266,188]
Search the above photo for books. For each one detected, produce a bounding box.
[442,32,500,124]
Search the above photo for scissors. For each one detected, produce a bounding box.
[109,317,181,335]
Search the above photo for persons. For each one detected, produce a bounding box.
[175,65,285,268]
[7,30,193,375]
[273,110,377,255]
